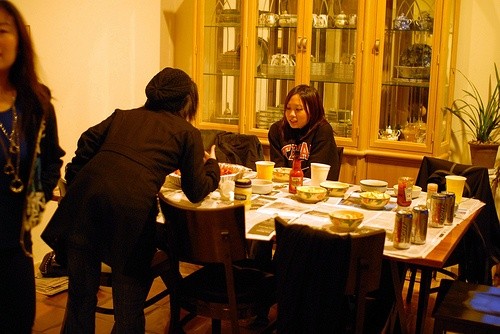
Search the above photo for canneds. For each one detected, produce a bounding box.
[430,193,446,227]
[393,209,413,250]
[234,178,252,211]
[443,193,455,225]
[410,206,428,245]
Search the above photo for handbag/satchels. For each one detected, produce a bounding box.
[24,192,44,230]
[39,251,68,278]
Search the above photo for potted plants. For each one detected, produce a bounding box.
[441,61,500,169]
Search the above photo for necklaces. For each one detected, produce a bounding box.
[0,97,25,193]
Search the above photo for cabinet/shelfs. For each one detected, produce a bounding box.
[192,0,461,158]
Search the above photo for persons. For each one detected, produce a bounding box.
[267,85,339,181]
[0,0,66,334]
[40,67,221,334]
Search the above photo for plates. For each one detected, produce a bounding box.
[400,43,432,66]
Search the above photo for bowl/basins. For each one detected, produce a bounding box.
[330,210,363,230]
[359,179,388,193]
[359,191,391,209]
[392,184,422,199]
[168,164,245,187]
[393,64,430,80]
[250,179,272,195]
[254,104,287,131]
[295,186,327,203]
[320,181,350,198]
[272,167,292,181]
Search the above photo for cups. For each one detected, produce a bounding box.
[256,161,275,182]
[311,163,331,187]
[219,180,234,202]
[446,175,467,205]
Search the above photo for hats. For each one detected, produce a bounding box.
[145,67,191,105]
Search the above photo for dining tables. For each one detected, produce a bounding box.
[157,163,485,334]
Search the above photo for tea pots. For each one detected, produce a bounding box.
[254,8,359,66]
[392,12,433,31]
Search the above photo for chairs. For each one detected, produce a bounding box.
[97,129,500,334]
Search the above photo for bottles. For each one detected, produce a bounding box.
[289,151,303,194]
[379,125,402,143]
[426,183,438,208]
[397,176,414,211]
[224,103,230,117]
